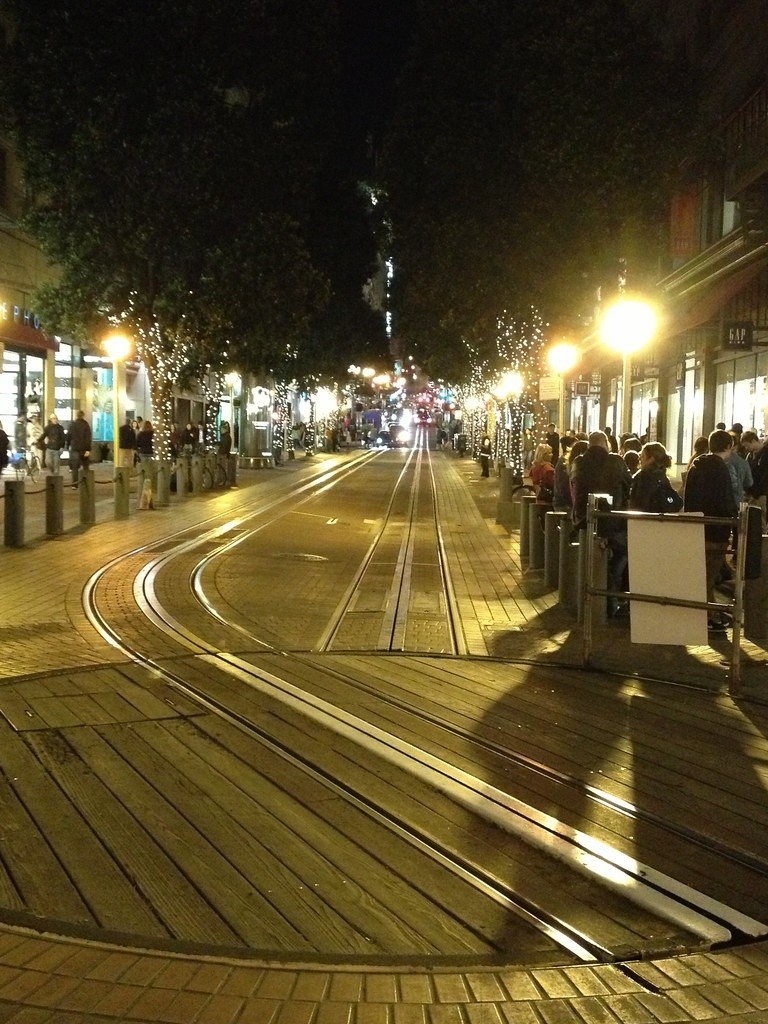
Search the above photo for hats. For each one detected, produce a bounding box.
[221,425,229,431]
[49,414,56,418]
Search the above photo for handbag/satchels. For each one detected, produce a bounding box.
[644,485,683,512]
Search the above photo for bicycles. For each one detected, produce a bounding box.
[166,443,228,492]
[14,445,43,485]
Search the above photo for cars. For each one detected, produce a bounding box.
[363,397,444,448]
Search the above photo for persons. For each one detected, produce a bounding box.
[25,416,44,468]
[524,428,534,469]
[37,414,66,475]
[66,410,91,490]
[477,432,492,476]
[436,426,448,446]
[531,423,768,633]
[292,408,378,453]
[14,413,30,455]
[451,429,458,450]
[0,421,12,474]
[120,416,239,476]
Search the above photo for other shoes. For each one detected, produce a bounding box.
[71,486,78,490]
[708,621,724,632]
[721,614,733,627]
[69,469,73,473]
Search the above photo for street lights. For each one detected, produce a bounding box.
[546,343,575,438]
[225,372,238,452]
[99,331,133,468]
[603,300,645,438]
